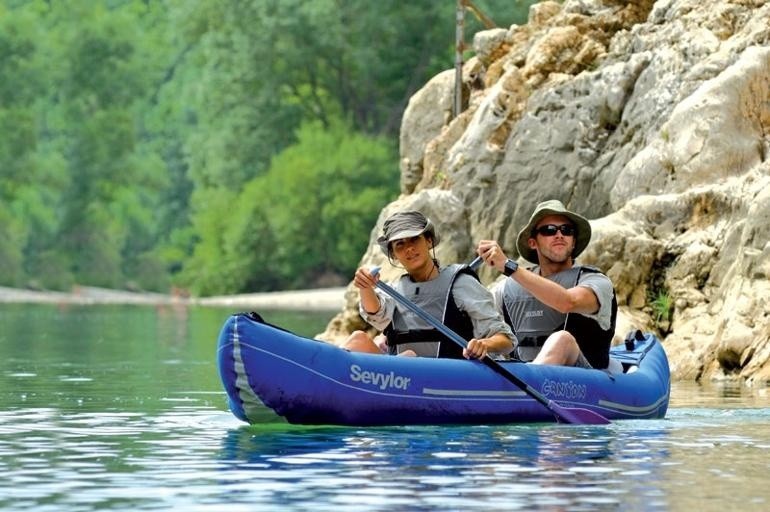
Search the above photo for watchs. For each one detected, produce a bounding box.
[502,259,518,277]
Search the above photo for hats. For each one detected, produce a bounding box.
[516,199,593,263]
[376,211,441,257]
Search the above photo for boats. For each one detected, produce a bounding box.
[217,313,676,422]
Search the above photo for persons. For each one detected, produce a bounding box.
[343,210,520,363]
[476,199,618,370]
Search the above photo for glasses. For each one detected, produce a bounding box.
[534,222,575,238]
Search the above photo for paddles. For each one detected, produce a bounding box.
[367,267,609,425]
